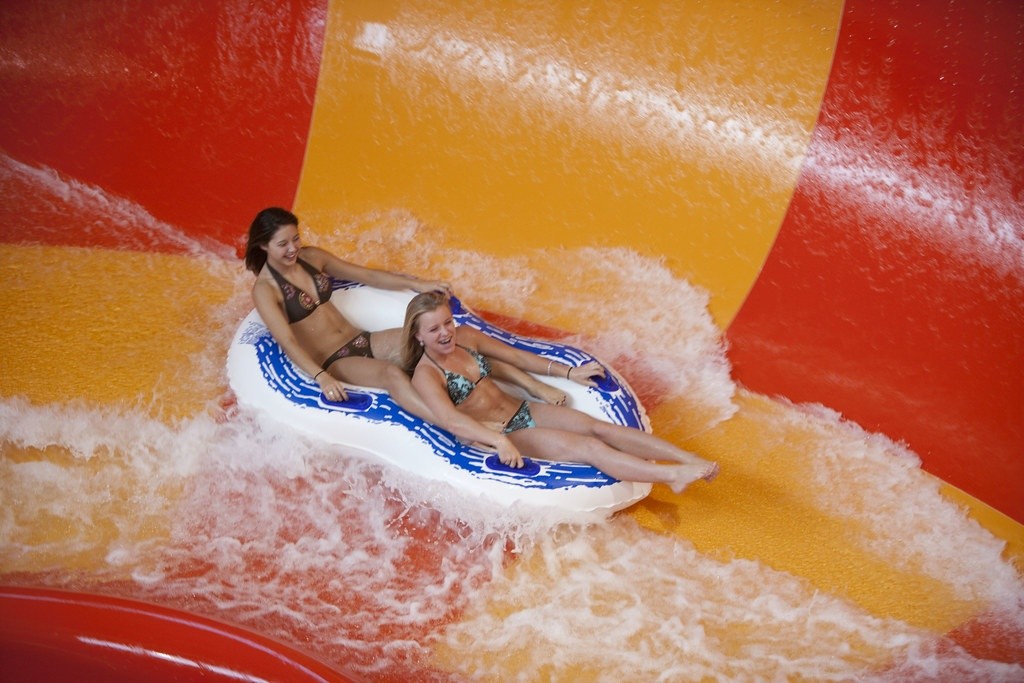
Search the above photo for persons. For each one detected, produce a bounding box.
[245,207,567,447]
[399,290,721,495]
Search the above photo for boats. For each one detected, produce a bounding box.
[223,275,657,529]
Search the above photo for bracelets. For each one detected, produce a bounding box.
[314,369,326,380]
[567,366,573,380]
[548,360,555,376]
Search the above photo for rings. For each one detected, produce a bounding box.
[329,390,334,397]
